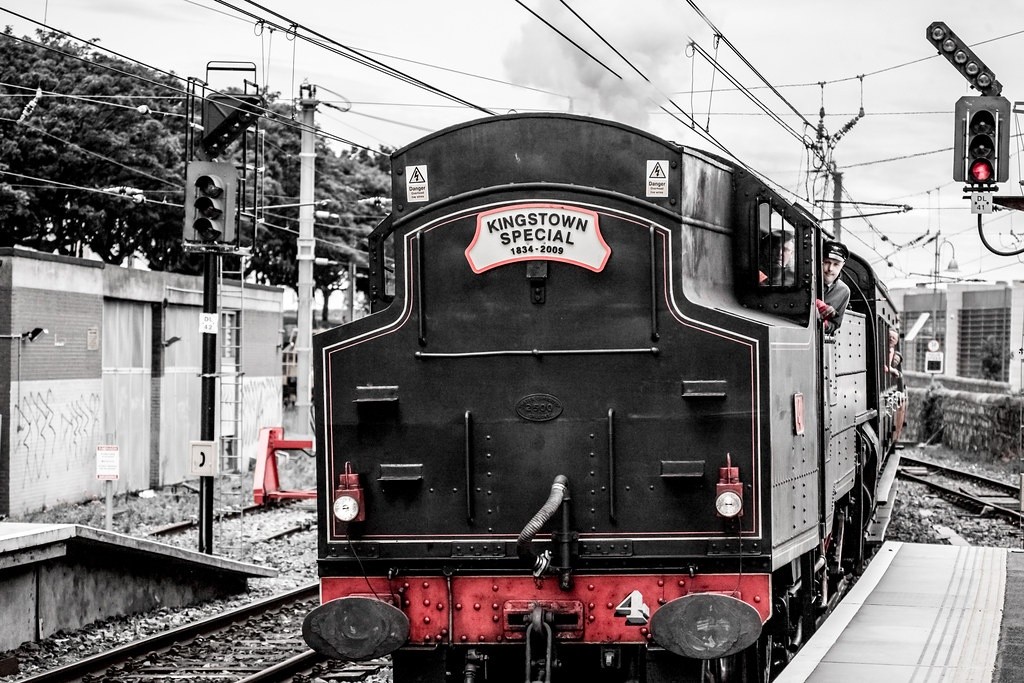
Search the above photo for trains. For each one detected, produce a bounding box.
[300,110,912,683]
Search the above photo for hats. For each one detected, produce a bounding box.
[823,242,849,262]
[894,351,903,363]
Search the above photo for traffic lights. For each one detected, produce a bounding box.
[193,98,264,157]
[185,161,236,244]
[931,25,997,91]
[953,96,1011,184]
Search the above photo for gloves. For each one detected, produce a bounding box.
[816,299,835,319]
[824,320,835,334]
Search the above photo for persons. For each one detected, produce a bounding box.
[816,241,850,332]
[884,328,903,378]
[759,230,795,287]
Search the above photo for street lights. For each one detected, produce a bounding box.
[932,241,959,340]
